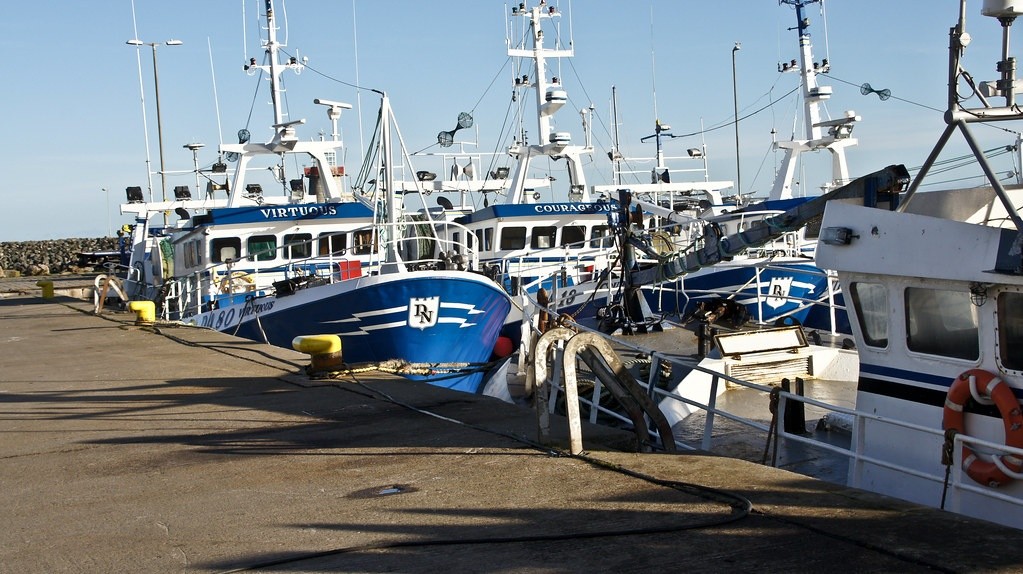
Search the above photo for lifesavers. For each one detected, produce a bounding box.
[223,271,256,294]
[941,369,1023,489]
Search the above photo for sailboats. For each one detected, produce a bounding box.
[118,0,514,394]
[395,1,1023,530]
